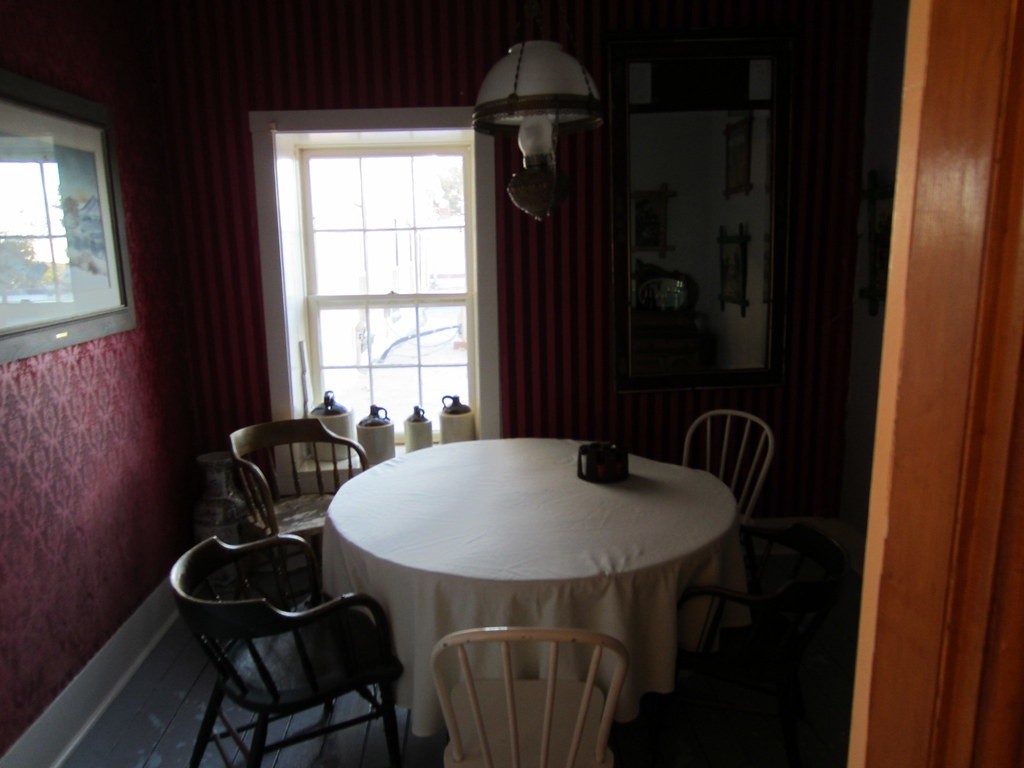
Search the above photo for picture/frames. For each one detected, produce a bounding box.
[632,189,676,252]
[722,116,754,200]
[716,223,751,317]
[0,68,137,365]
[858,172,894,316]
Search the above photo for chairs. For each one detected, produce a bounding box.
[682,409,774,559]
[229,417,369,609]
[646,521,849,768]
[429,626,629,768]
[169,531,405,768]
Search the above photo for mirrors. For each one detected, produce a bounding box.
[601,24,790,392]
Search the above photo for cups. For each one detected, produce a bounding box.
[577,442,629,483]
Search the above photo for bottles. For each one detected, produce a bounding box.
[439,395,474,444]
[356,405,395,465]
[311,391,356,461]
[405,406,433,453]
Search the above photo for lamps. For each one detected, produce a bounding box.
[475,0,604,223]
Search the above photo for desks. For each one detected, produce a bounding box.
[323,438,751,737]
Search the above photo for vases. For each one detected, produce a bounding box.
[193,451,247,600]
